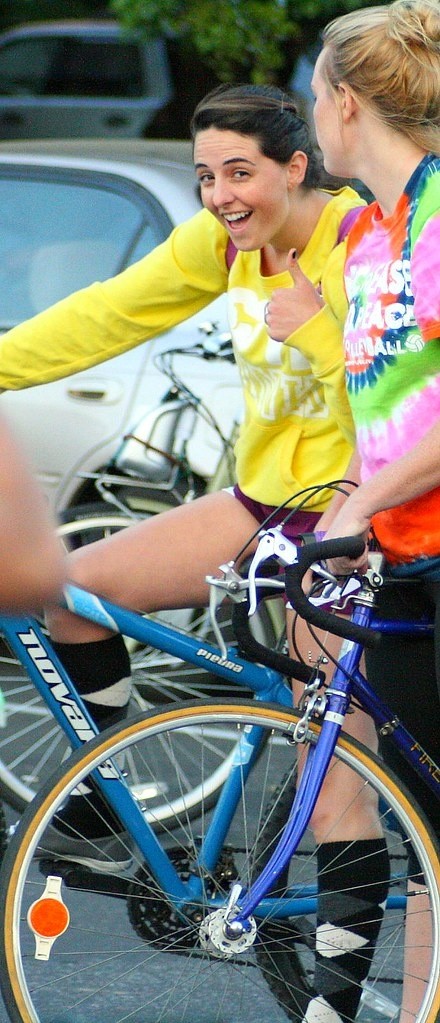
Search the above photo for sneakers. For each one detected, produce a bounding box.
[6,820,134,873]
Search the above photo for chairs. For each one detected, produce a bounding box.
[29,240,117,311]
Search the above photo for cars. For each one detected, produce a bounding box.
[2,21,171,140]
[1,151,240,705]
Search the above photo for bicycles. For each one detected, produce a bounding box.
[1,321,278,837]
[0,481,439,1021]
[0,580,407,1022]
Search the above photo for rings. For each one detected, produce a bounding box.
[264,302,271,324]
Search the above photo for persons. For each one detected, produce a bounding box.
[2,86,390,1023]
[0,414,73,613]
[312,1,440,1023]
